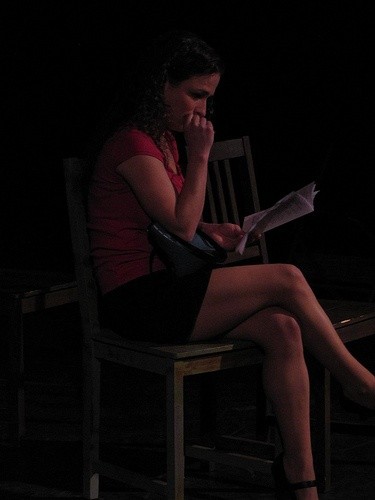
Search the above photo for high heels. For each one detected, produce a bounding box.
[272,452,318,500]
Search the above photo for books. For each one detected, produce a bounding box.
[234,180,316,257]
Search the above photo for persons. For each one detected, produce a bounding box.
[84,27,375,499]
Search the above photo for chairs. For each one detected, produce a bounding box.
[184,135,374,489]
[1,254,85,445]
[63,154,298,500]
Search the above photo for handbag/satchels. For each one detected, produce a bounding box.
[147,223,226,283]
